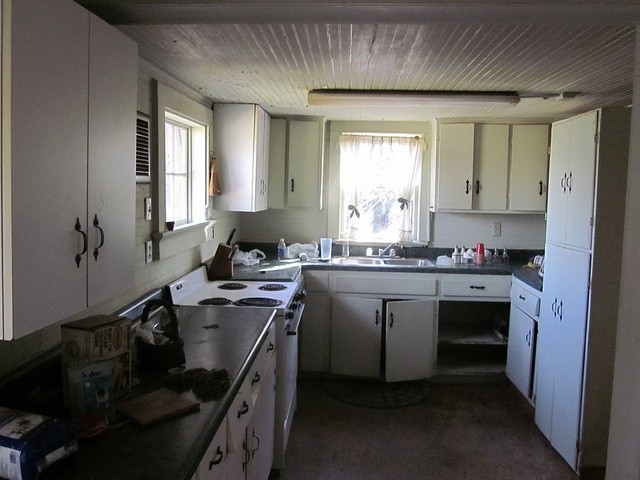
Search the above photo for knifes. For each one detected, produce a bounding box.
[229,244,239,260]
[226,228,236,245]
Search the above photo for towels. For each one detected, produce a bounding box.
[436,255,454,265]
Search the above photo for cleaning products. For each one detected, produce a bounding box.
[342,232,350,257]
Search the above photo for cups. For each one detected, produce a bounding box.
[166,221,175,231]
[484,249,491,258]
[342,241,350,257]
[160,308,179,331]
[320,237,332,259]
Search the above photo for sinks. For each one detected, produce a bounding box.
[331,255,384,268]
[383,257,434,268]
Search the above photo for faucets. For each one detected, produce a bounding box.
[400,246,407,258]
[378,242,398,257]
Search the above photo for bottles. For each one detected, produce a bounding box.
[277,238,287,260]
[501,248,510,262]
[452,244,461,264]
[460,244,467,264]
[492,247,501,263]
[477,243,484,265]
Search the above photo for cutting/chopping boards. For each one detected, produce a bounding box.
[114,387,200,429]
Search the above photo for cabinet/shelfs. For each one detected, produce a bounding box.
[0,0,138,342]
[286,120,320,210]
[259,322,276,479]
[437,273,513,377]
[546,110,598,250]
[510,124,549,212]
[331,271,438,383]
[535,244,591,472]
[227,354,258,479]
[300,269,331,374]
[212,102,271,212]
[437,124,510,211]
[195,417,227,479]
[505,283,542,409]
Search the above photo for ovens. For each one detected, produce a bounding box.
[272,302,307,478]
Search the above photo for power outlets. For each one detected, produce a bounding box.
[145,197,152,220]
[146,240,153,262]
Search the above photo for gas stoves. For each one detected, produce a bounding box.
[173,279,299,316]
